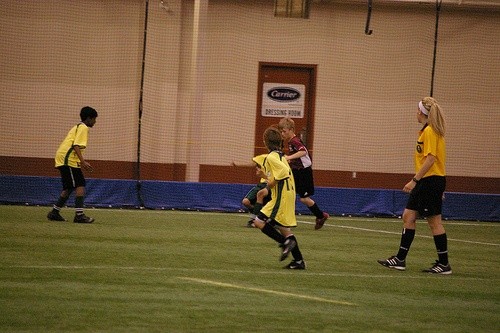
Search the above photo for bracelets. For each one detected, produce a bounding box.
[412,177,420,184]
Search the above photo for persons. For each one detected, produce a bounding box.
[47,106,98,223]
[377,97,452,275]
[279,118,329,229]
[242,124,304,269]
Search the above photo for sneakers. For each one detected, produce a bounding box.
[315,212,329,230]
[422,259,453,275]
[278,239,296,261]
[377,255,406,271]
[282,260,305,270]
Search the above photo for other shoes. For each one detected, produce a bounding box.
[247,218,257,228]
[73,213,95,224]
[47,210,68,222]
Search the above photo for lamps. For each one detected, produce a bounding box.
[159,1,170,12]
